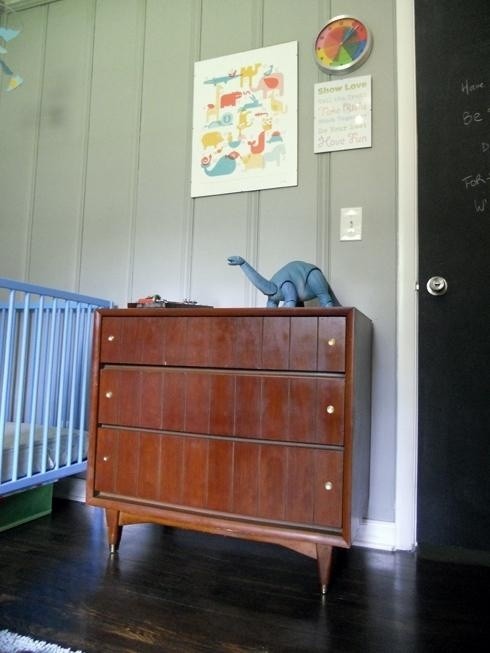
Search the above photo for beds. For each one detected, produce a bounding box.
[0,280,115,499]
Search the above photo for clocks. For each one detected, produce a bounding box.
[313,15,373,75]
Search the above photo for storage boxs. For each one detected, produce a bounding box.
[1,478,60,533]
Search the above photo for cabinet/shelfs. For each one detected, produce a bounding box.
[84,306,374,598]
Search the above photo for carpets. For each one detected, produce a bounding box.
[0,629,86,653]
